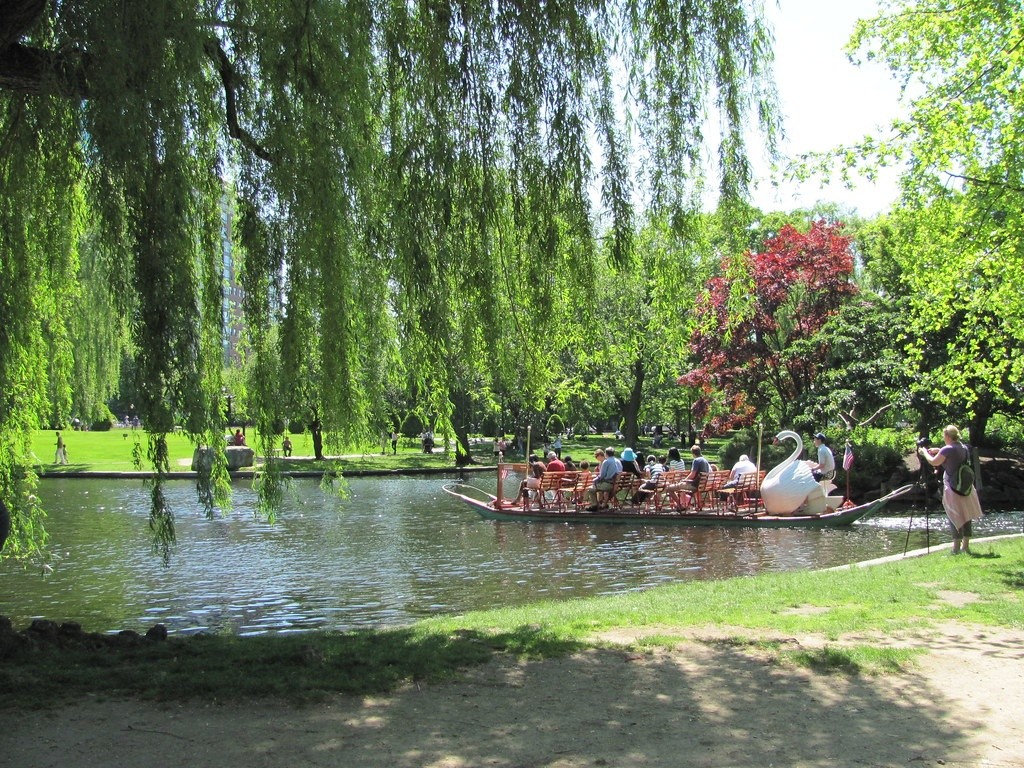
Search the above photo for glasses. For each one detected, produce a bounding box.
[595,455,600,459]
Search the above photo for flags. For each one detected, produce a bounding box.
[843,443,855,471]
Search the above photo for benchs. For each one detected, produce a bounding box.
[273,449,293,457]
[523,470,766,516]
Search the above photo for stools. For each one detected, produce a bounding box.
[819,469,836,514]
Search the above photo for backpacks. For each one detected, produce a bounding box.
[946,448,975,497]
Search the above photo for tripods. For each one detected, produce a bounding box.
[903,459,929,557]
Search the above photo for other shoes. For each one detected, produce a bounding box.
[684,494,691,508]
[940,548,972,558]
[623,497,633,505]
[511,497,584,509]
[587,501,609,512]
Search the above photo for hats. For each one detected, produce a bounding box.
[621,447,637,461]
[813,433,826,441]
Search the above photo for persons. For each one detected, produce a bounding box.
[810,433,835,483]
[543,434,561,460]
[419,428,435,450]
[282,437,293,457]
[62,444,69,463]
[585,447,622,512]
[392,432,400,455]
[667,445,712,511]
[234,429,248,446]
[54,431,65,464]
[133,415,139,430]
[511,446,718,513]
[681,428,705,451]
[721,454,756,508]
[493,431,524,457]
[918,425,983,558]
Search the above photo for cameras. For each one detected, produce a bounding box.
[916,437,932,449]
